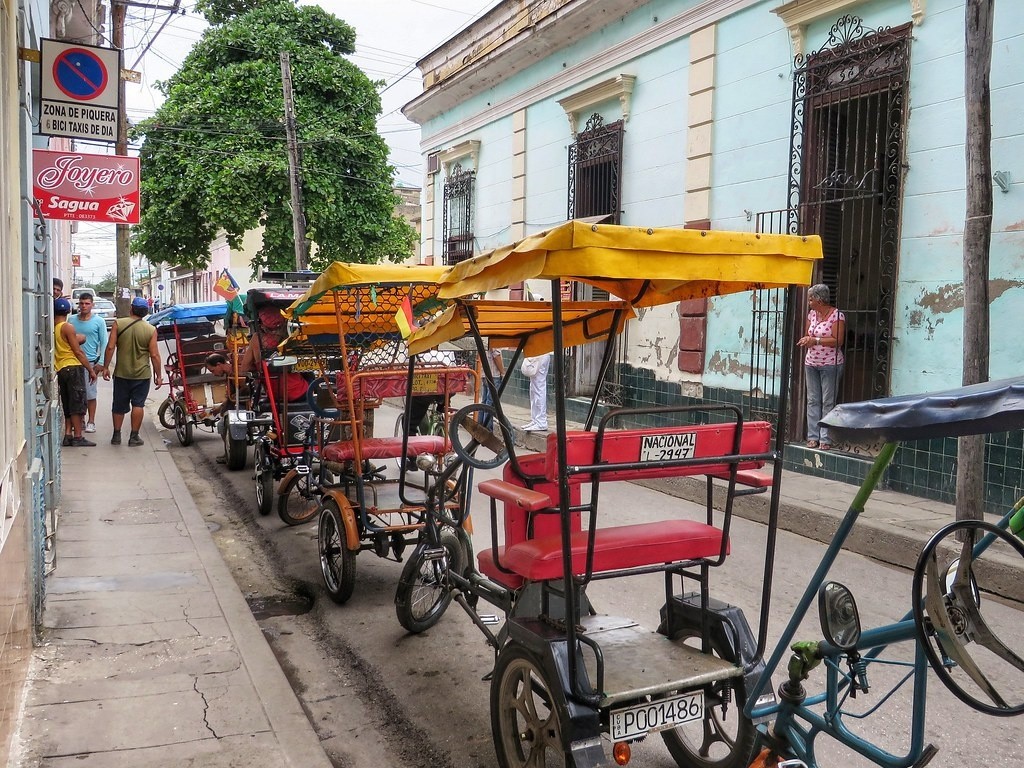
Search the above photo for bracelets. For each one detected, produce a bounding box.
[816,337,821,345]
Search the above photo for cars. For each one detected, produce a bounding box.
[70,288,96,309]
[89,296,117,327]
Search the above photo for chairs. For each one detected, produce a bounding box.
[478,454,582,590]
[180,340,201,376]
[327,402,375,474]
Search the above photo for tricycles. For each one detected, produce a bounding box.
[388,219,824,768]
[222,291,273,474]
[145,302,230,446]
[739,377,1024,766]
[277,260,484,609]
[241,284,358,515]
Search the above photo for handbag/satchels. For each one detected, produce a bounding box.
[521,358,539,376]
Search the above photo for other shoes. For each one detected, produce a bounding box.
[128,431,144,446]
[111,430,121,445]
[819,444,830,449]
[807,440,817,448]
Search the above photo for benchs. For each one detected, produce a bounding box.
[480,422,773,579]
[324,371,469,462]
[260,396,319,411]
[181,335,227,366]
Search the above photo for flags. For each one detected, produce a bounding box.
[394,286,421,340]
[213,268,240,301]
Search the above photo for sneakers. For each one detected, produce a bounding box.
[523,422,547,430]
[72,421,86,430]
[72,437,97,446]
[85,422,96,433]
[63,434,73,446]
[521,421,535,429]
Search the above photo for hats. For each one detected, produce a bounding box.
[54,298,70,311]
[131,297,148,309]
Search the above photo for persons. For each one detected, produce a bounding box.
[796,284,845,451]
[104,297,162,446]
[204,354,247,464]
[145,295,176,314]
[52,278,64,299]
[401,347,458,472]
[68,294,110,432]
[477,347,505,431]
[521,294,550,431]
[241,319,316,404]
[53,299,97,447]
